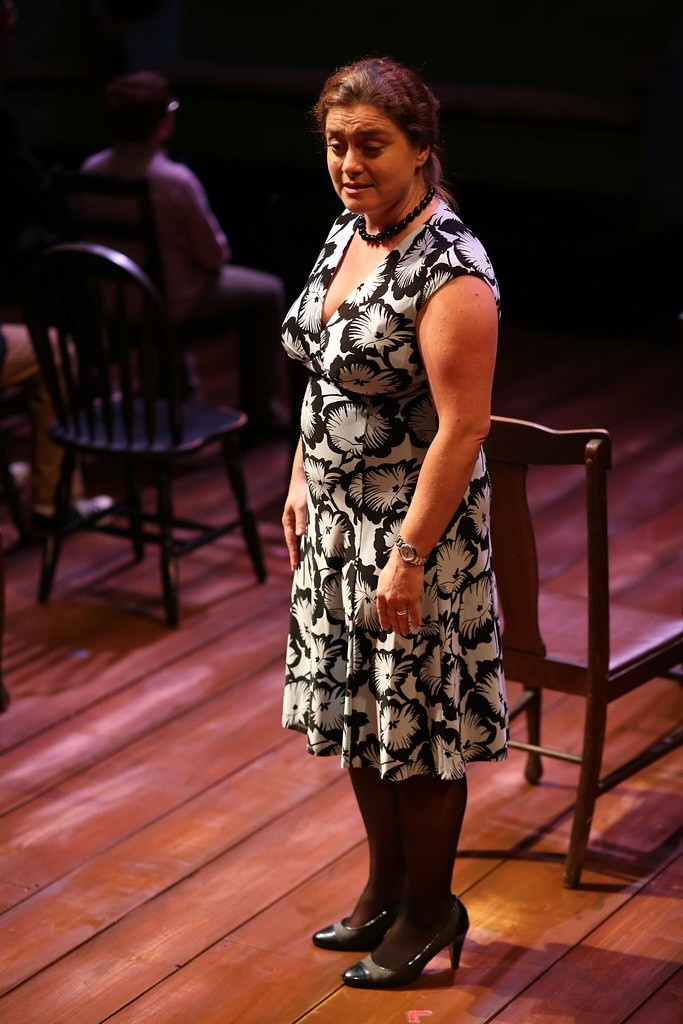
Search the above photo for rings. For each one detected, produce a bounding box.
[397,610,408,616]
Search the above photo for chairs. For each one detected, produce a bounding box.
[0,383,38,547]
[22,241,270,629]
[49,171,279,477]
[484,415,683,890]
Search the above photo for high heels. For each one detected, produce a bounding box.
[343,894,470,989]
[312,905,397,951]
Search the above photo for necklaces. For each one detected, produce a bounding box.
[357,186,436,242]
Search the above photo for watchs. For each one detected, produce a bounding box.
[394,533,429,566]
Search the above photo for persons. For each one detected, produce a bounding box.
[81,73,303,445]
[280,58,513,990]
[0,317,117,533]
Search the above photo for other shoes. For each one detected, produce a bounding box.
[34,497,117,544]
